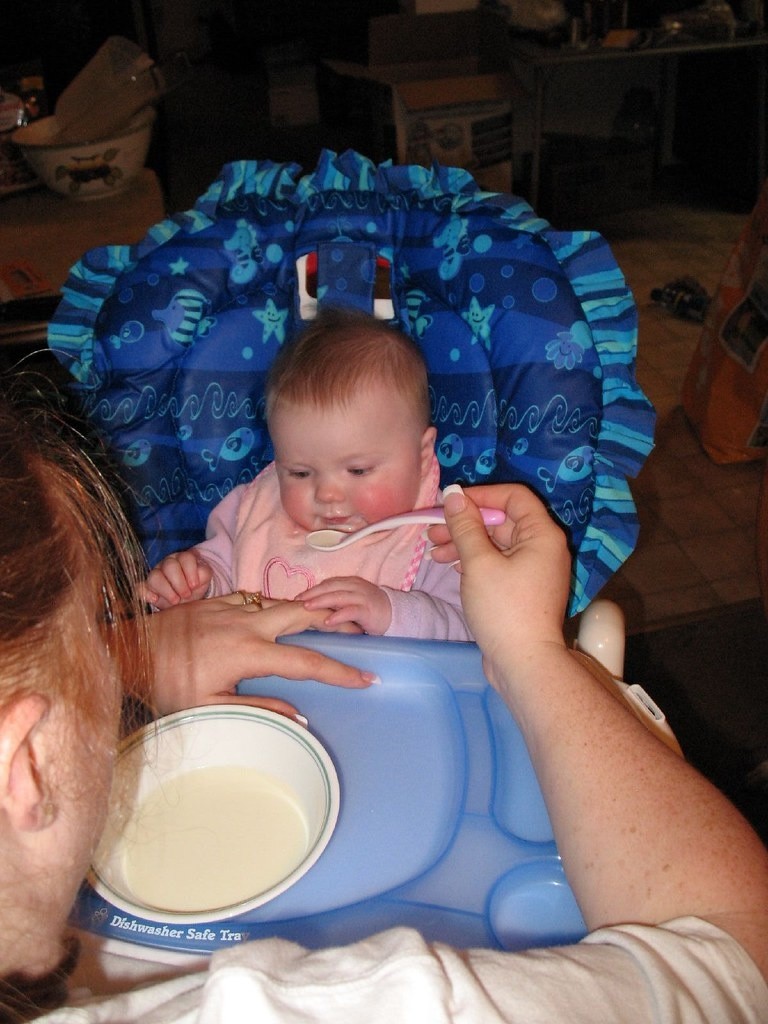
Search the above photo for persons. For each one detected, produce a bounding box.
[137,308,476,641]
[0,372,768,1024]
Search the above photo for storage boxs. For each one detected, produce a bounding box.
[316,5,511,196]
[523,137,652,225]
[266,63,322,130]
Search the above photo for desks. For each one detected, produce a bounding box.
[522,32,768,209]
[0,166,165,305]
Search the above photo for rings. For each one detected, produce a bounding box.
[233,591,264,611]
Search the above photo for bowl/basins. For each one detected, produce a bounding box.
[12,105,154,200]
[83,705,338,925]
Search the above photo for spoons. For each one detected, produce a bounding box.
[305,508,507,552]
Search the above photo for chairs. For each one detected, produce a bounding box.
[43,146,657,616]
